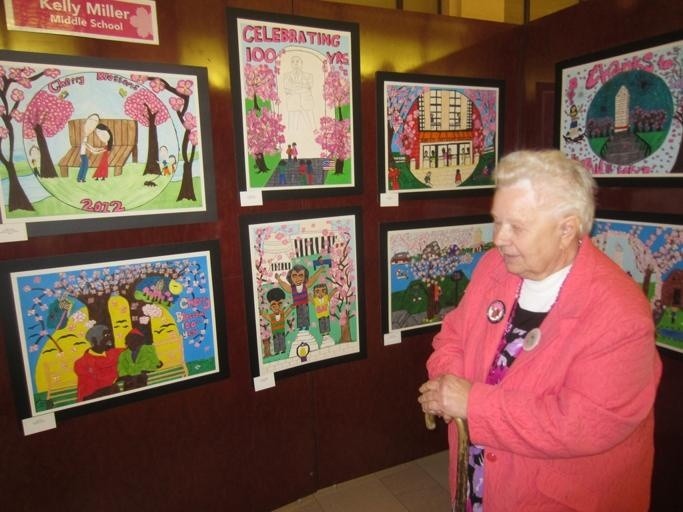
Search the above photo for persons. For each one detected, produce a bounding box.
[416,149,663,512]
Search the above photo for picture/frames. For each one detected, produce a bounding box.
[554,28,682,188]
[0,49,217,245]
[225,8,364,209]
[0,239,231,437]
[379,216,493,348]
[375,70,505,209]
[238,206,367,392]
[589,211,682,363]
